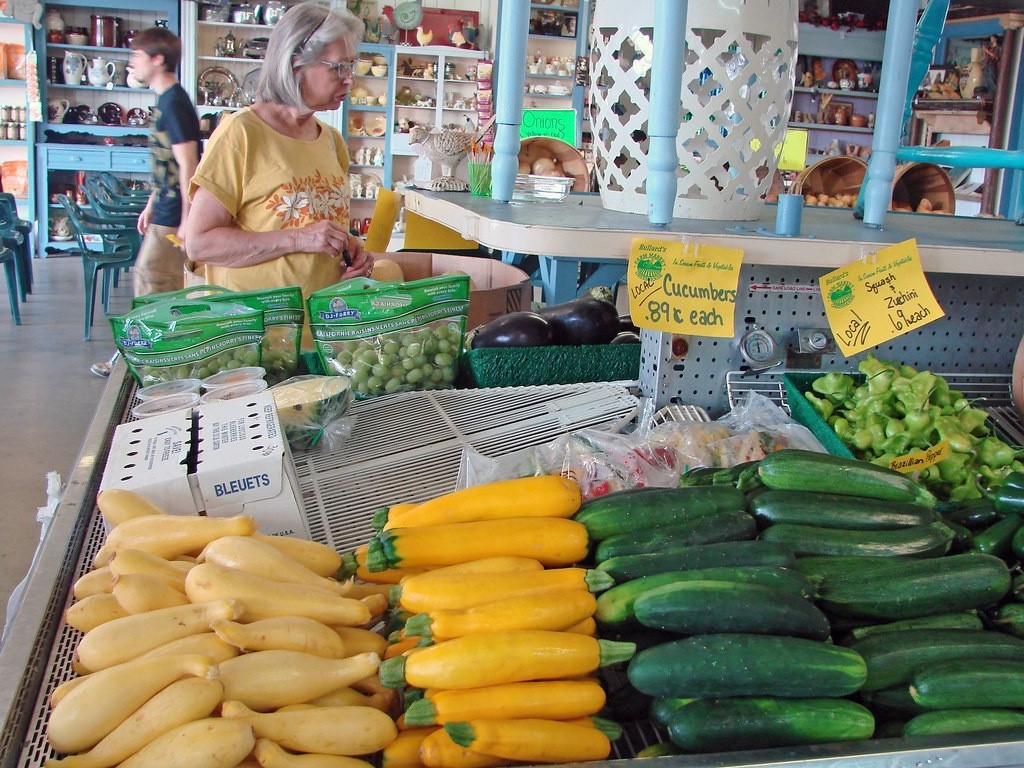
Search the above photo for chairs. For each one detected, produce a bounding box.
[0,171,154,340]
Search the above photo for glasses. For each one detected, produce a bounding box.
[320,59,357,80]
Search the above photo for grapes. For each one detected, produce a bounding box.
[139,339,294,388]
[331,325,464,400]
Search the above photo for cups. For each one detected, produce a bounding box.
[352,88,377,106]
[109,58,128,85]
[467,163,492,197]
[856,74,869,89]
[775,194,804,236]
[213,43,225,57]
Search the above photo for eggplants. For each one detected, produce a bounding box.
[464,285,641,352]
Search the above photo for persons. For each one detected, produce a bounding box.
[186,3,375,350]
[90,27,204,378]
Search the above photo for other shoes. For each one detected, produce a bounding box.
[91,363,110,379]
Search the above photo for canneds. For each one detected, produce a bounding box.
[0,105,26,141]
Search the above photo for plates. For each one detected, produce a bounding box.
[66,103,148,126]
[366,116,386,136]
[198,66,262,105]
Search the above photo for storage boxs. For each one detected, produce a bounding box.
[96,391,312,542]
[365,251,531,330]
[779,371,866,460]
[466,343,640,388]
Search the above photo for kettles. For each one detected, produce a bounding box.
[231,0,262,26]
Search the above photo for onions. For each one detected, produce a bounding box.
[516,157,566,178]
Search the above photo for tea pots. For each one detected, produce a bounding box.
[82,56,149,88]
[47,98,69,123]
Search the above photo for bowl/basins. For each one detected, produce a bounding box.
[851,114,866,127]
[244,37,269,59]
[131,367,268,421]
[354,54,387,76]
[840,79,855,89]
[519,136,590,194]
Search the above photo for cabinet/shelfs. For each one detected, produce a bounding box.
[0,0,1023,258]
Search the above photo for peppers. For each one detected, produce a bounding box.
[803,352,1024,503]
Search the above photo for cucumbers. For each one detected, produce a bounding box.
[578,449,1024,756]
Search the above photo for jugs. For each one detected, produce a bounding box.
[217,30,243,56]
[63,50,88,84]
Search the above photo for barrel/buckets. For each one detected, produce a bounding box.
[90,14,122,48]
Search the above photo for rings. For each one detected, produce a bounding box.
[367,269,372,276]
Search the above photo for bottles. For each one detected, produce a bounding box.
[527,47,574,76]
[46,9,89,45]
[124,30,140,47]
[805,72,812,87]
[0,105,27,140]
[445,63,455,78]
[869,112,874,127]
[794,111,801,122]
[582,98,593,143]
[419,62,437,79]
